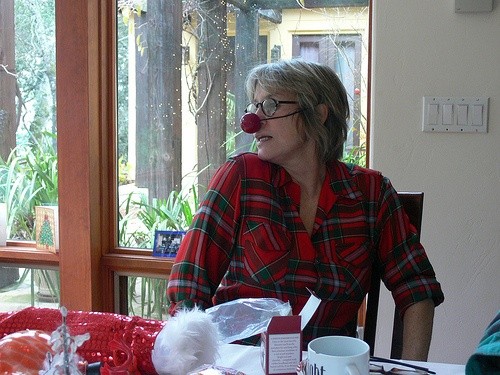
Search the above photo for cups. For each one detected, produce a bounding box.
[307,335,370,375]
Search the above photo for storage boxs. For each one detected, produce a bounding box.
[260,294,322,375]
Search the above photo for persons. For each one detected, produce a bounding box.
[166,59,444,362]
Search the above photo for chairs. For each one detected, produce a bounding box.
[354,191,424,360]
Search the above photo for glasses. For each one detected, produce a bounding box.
[244,98,303,116]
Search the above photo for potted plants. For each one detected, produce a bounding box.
[0,118,60,310]
